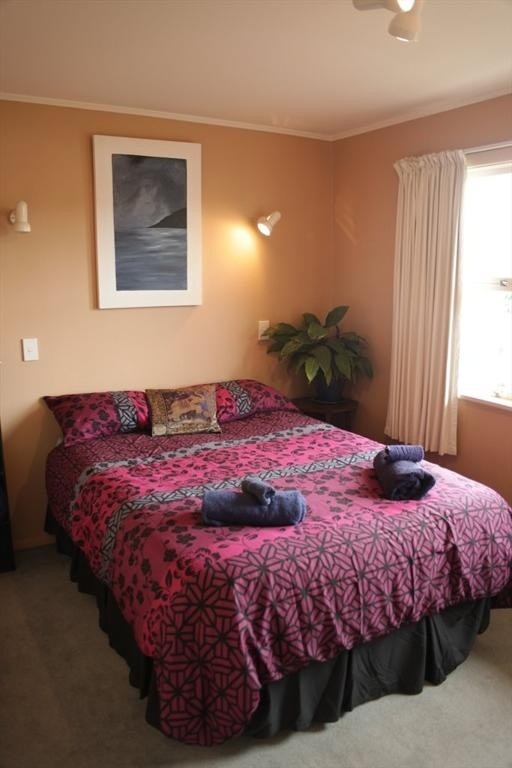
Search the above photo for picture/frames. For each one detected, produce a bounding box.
[92,135,204,309]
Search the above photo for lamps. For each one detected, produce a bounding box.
[388,0,422,41]
[353,0,415,14]
[257,209,282,236]
[9,201,31,233]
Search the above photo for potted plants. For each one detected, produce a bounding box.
[260,305,374,404]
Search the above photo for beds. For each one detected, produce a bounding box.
[43,410,512,747]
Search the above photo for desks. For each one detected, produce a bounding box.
[289,396,359,432]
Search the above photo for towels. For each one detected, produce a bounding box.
[373,444,436,500]
[201,477,306,525]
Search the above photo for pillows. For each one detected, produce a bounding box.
[145,385,222,437]
[186,379,300,423]
[41,391,150,448]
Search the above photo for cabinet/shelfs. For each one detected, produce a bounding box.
[0,418,18,573]
